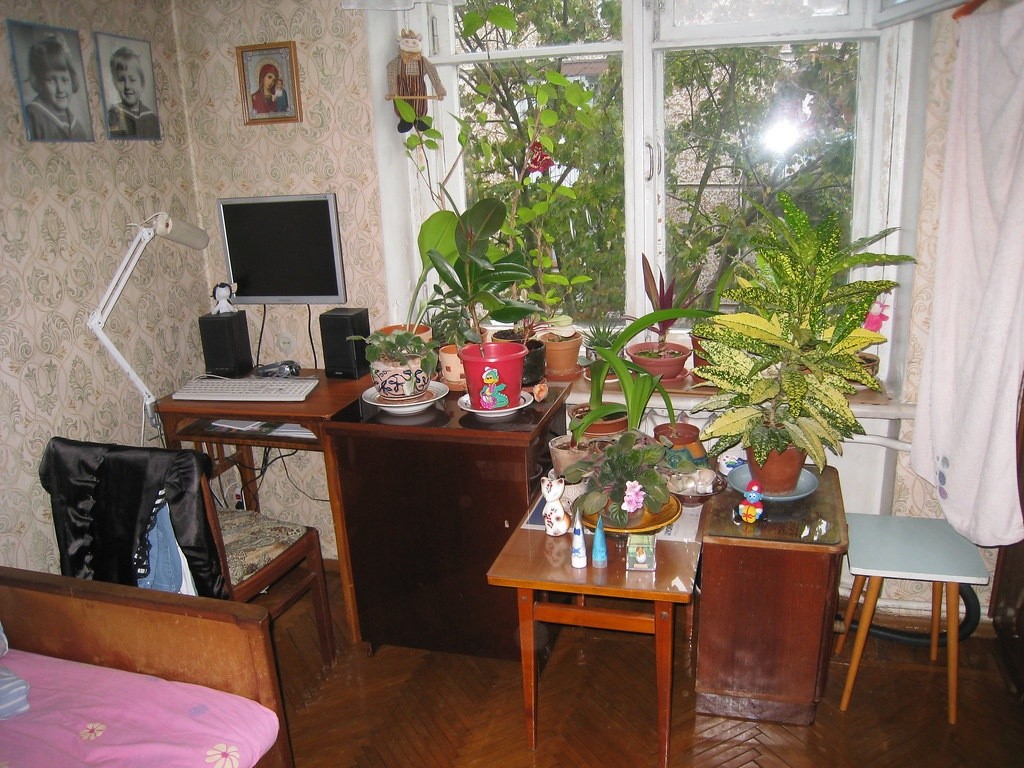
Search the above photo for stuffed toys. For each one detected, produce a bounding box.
[386,29,446,132]
[211,283,237,314]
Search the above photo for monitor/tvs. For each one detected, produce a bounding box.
[217,193,347,304]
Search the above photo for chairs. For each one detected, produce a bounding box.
[37,436,338,667]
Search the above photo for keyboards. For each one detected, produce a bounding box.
[172,378,321,401]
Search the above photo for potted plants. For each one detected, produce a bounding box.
[344,6,919,527]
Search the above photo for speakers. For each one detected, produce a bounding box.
[319,307,371,380]
[199,310,253,378]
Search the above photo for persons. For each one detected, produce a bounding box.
[734,480,767,523]
[25,39,88,141]
[107,47,161,139]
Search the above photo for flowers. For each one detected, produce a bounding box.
[563,433,674,528]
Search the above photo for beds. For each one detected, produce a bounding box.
[0,566,296,768]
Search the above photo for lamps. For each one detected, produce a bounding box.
[86,212,209,438]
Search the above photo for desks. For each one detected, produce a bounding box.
[151,369,376,645]
[486,453,719,768]
[835,513,990,725]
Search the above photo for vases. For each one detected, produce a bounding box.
[604,498,644,521]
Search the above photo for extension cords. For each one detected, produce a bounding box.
[233,488,246,511]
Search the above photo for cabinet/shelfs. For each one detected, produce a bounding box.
[693,466,849,726]
[321,380,573,663]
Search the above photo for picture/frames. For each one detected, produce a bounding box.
[236,42,303,126]
[94,30,161,141]
[7,20,94,143]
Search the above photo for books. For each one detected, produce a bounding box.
[211,419,266,431]
